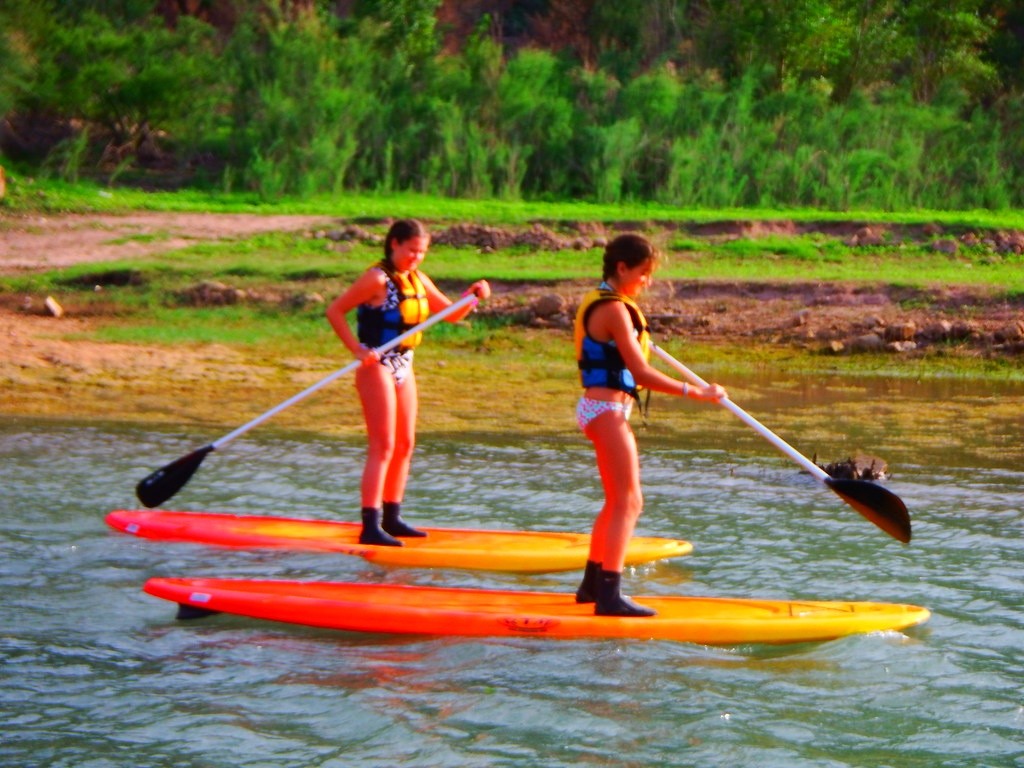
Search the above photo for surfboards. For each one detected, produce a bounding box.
[141,579,935,642]
[104,509,692,572]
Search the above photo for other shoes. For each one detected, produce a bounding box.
[575,581,595,602]
[360,528,403,546]
[381,521,427,537]
[595,592,656,616]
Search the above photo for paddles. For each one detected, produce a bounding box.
[643,332,914,543]
[134,288,483,509]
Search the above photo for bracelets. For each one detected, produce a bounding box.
[682,382,688,400]
[355,343,370,360]
[462,290,481,309]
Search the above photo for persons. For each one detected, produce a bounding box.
[574,234,727,617]
[326,218,492,547]
